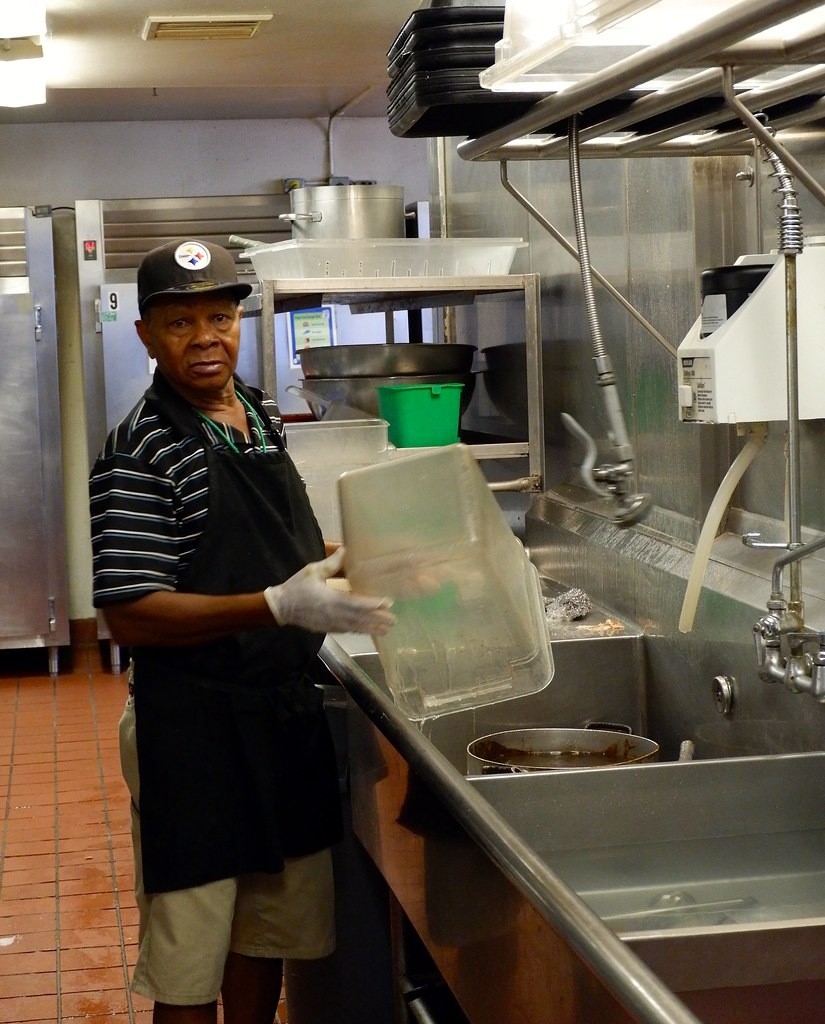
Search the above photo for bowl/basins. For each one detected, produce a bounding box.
[299,370,478,421]
[295,343,478,377]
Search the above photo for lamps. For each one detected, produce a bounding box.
[0,0,48,109]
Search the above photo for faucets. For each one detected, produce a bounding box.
[588,460,651,526]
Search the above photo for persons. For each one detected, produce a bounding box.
[90,239,399,1024]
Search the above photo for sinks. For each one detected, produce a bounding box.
[467,755,824,945]
[351,633,824,792]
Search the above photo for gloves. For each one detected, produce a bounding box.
[264,546,397,636]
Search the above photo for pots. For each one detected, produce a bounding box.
[279,184,414,240]
[466,722,660,775]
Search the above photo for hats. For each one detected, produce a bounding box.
[137,238,252,313]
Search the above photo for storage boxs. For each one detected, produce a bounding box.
[375,384,465,447]
[479,0,825,92]
[337,442,555,723]
[238,237,529,277]
